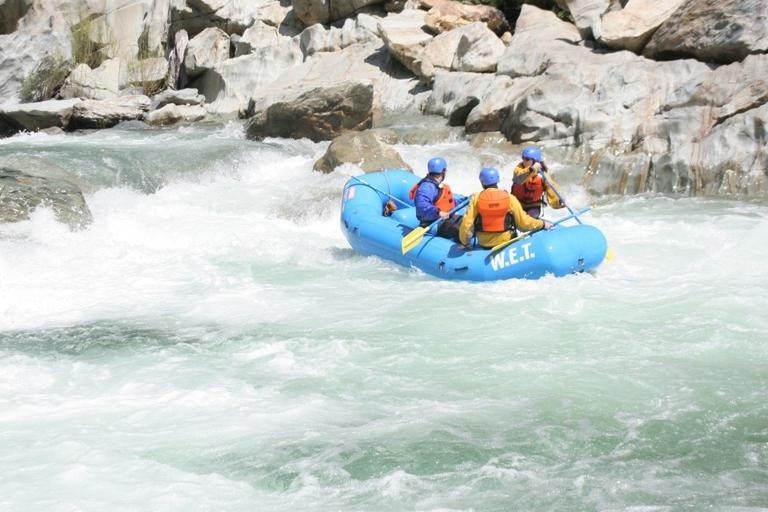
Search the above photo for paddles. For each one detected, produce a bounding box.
[491,204,596,252]
[539,169,614,263]
[402,195,471,255]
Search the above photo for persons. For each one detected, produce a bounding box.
[510,146,568,220]
[459,168,554,250]
[415,157,464,243]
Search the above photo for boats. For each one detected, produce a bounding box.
[341,170,608,282]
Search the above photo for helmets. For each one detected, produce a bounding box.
[426,156,448,175]
[477,167,501,187]
[521,146,544,163]
